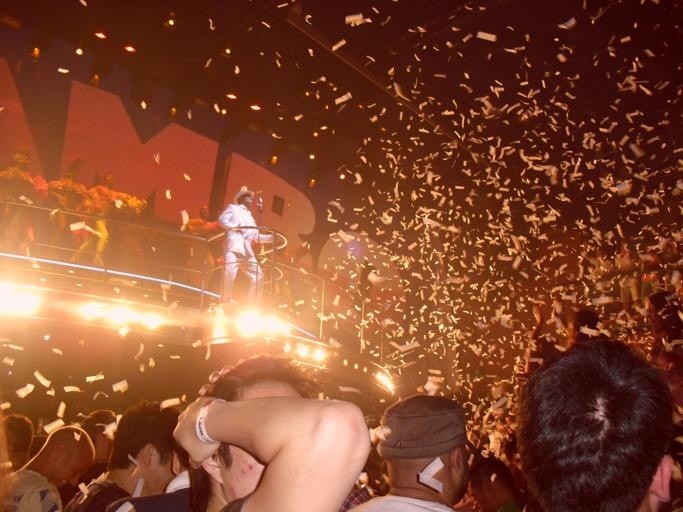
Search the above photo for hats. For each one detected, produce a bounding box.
[377,394,468,458]
[233,191,254,202]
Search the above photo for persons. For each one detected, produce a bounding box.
[1,152,222,299]
[1,291,683,512]
[216,184,282,306]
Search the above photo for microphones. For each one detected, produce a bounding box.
[256,196,263,212]
